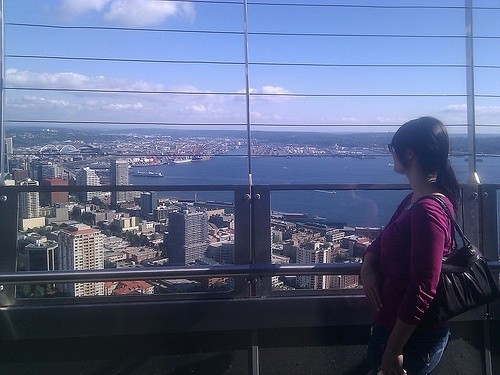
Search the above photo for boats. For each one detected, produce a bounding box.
[130,169,165,177]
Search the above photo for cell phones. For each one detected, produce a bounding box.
[377,369,407,375]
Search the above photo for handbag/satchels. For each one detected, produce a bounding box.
[409,194,500,321]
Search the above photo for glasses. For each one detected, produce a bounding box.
[389,144,407,152]
